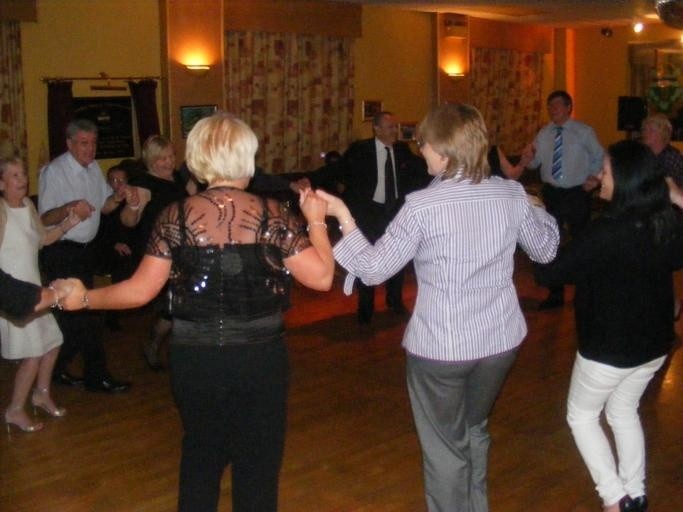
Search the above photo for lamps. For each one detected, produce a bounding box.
[186,64,209,79]
[447,72,464,84]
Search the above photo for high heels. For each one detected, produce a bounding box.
[31,391,68,418]
[4,407,43,433]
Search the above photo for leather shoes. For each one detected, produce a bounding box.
[52,371,83,386]
[81,367,131,394]
[143,344,170,375]
[358,302,410,329]
[619,495,648,512]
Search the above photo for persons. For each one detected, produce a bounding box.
[57,111,335,512]
[300,103,561,512]
[0,269,74,321]
[325,151,353,196]
[249,171,301,194]
[37,119,133,394]
[522,91,608,310]
[0,157,80,433]
[119,134,198,272]
[94,166,146,332]
[532,139,683,512]
[487,143,536,181]
[302,112,434,327]
[591,112,683,321]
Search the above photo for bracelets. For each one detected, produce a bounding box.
[48,286,59,309]
[82,290,89,309]
[113,195,122,204]
[305,221,328,232]
[338,218,356,231]
[61,223,67,234]
[130,202,141,210]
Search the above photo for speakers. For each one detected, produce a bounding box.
[617,95,647,132]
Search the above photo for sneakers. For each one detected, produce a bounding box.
[537,297,565,311]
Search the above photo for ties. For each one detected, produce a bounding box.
[551,129,564,181]
[385,148,396,213]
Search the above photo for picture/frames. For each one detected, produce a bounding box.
[359,100,384,124]
[178,103,218,139]
[396,121,419,144]
